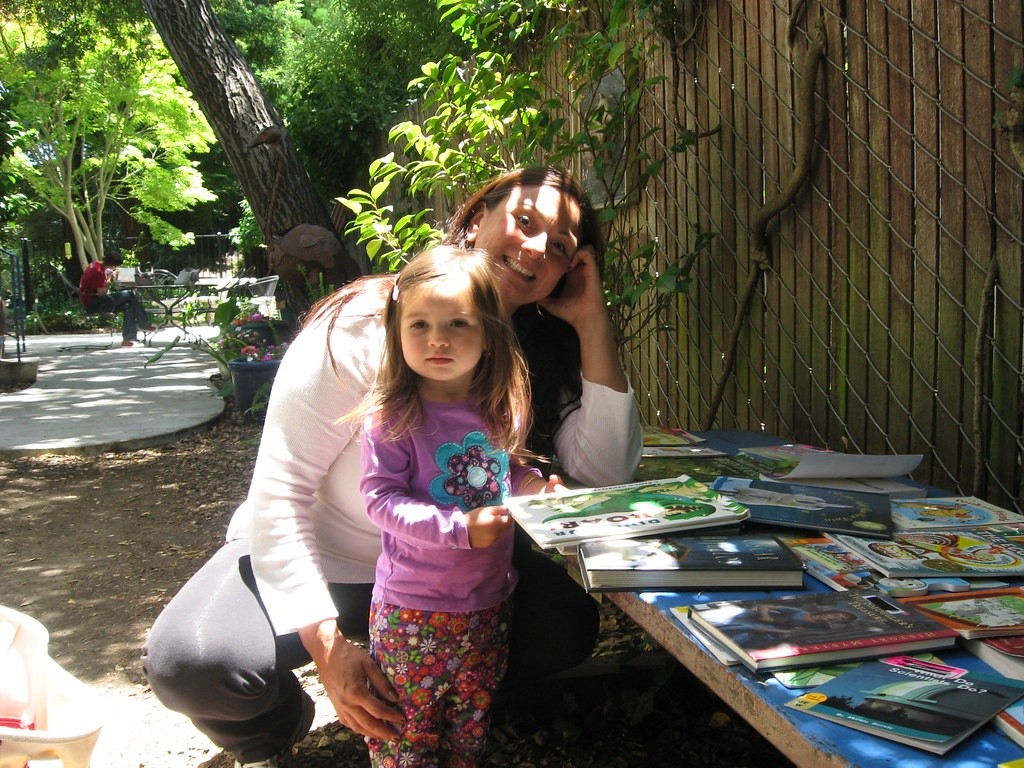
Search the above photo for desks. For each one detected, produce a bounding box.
[123,283,217,347]
[529,428,1023,767]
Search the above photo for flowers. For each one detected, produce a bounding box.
[218,312,290,362]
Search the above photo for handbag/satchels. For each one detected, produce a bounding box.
[174,268,199,292]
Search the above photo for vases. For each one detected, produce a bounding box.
[227,353,284,424]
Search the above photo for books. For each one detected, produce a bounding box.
[501,427,1024,768]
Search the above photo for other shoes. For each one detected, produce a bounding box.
[141,326,157,332]
[233,688,315,768]
[122,340,133,346]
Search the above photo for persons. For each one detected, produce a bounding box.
[80,251,165,347]
[148,167,644,768]
[365,246,565,768]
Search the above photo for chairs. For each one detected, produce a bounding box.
[192,277,240,327]
[56,270,117,352]
[218,275,279,300]
